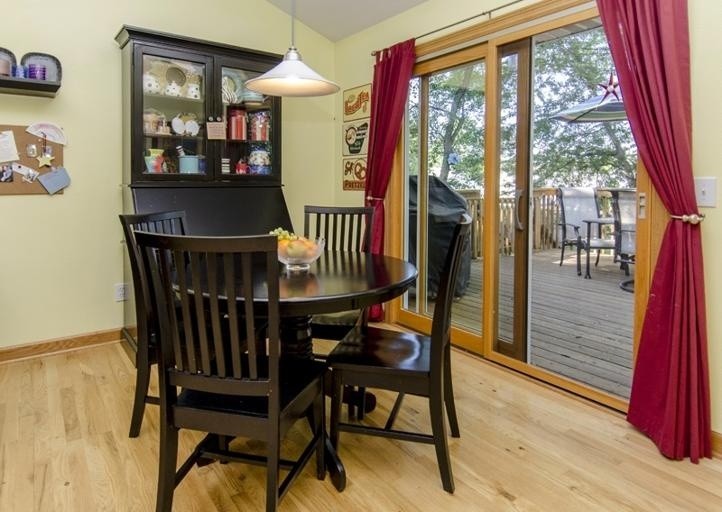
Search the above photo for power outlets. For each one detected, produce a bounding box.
[115,283,127,302]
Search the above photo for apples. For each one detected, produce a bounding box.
[279,277,318,298]
[279,239,316,260]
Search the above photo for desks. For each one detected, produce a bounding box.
[580,219,615,278]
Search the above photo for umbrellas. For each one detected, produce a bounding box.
[548,91,630,124]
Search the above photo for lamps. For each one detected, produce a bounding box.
[242,0,341,97]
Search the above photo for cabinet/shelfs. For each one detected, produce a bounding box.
[115,23,294,369]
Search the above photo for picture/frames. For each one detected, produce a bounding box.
[342,157,369,191]
[342,119,371,156]
[343,83,371,122]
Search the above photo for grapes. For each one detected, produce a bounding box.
[269,226,297,241]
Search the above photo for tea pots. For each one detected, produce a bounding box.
[233,155,251,176]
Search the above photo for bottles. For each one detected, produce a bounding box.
[143,71,201,100]
[144,113,170,135]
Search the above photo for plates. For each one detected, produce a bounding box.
[220,68,243,104]
[171,116,200,137]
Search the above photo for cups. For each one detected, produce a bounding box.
[0,58,46,81]
[222,157,231,175]
[248,149,273,175]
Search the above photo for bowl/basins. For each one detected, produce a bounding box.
[228,108,271,141]
[243,81,270,108]
[277,235,326,272]
[176,154,200,175]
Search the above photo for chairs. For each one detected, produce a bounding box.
[304,205,376,420]
[556,185,615,277]
[119,211,192,437]
[326,213,472,493]
[134,231,326,511]
[611,189,637,293]
